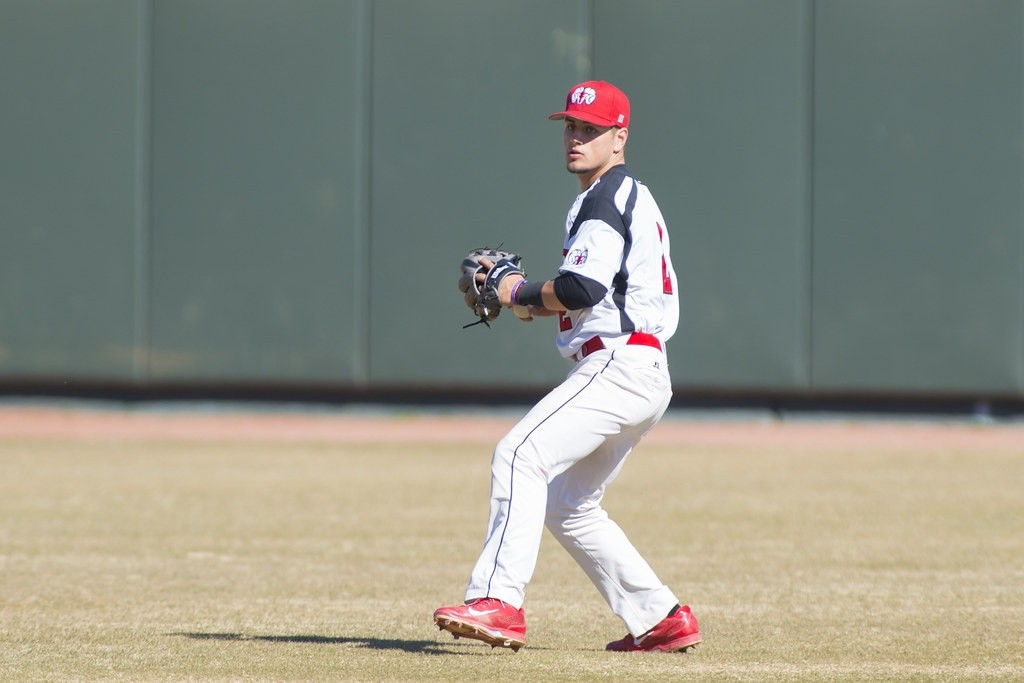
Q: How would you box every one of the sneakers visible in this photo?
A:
[605,604,702,651]
[433,597,526,652]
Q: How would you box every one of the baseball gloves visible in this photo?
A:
[459,248,520,321]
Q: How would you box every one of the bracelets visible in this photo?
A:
[511,280,548,308]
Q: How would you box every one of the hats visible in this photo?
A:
[546,77,630,128]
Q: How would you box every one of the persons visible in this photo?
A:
[434,80,703,655]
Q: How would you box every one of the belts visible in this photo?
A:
[570,333,662,360]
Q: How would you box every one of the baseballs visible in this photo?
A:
[512,304,534,318]
